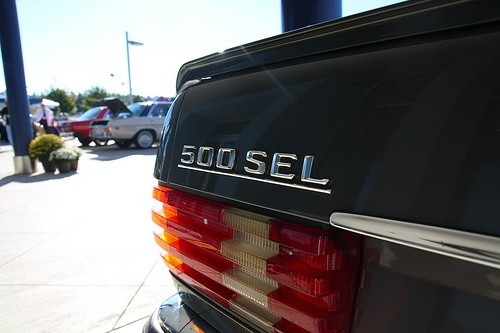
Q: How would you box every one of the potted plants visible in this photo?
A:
[28,134,83,173]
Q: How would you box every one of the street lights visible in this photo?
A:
[125,30,143,105]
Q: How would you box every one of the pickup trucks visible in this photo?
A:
[142,0,500,333]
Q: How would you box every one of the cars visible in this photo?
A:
[57,97,175,149]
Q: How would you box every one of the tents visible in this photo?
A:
[1,92,60,108]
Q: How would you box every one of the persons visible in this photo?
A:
[0,115,13,146]
[34,103,59,140]
